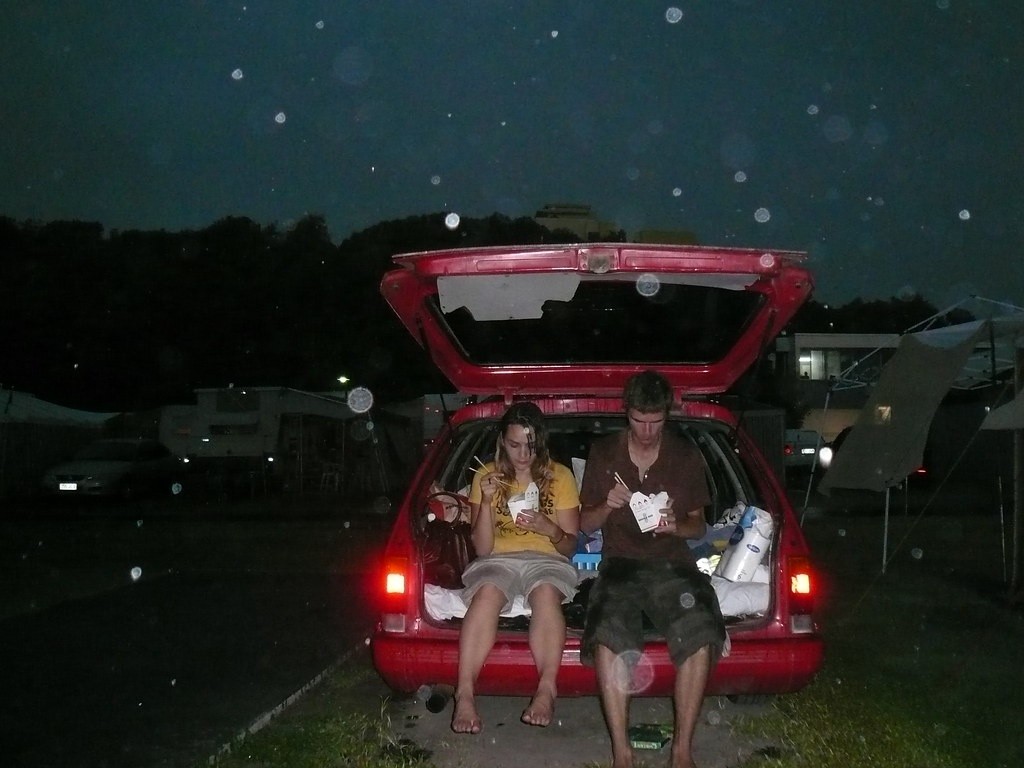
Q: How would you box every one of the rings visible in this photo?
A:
[489,479,491,484]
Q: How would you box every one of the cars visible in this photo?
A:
[365,247,825,715]
[781,428,828,482]
[826,424,928,488]
[42,433,170,503]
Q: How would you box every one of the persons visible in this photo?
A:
[580,371,724,768]
[451,402,580,732]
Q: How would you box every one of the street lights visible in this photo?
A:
[337,376,350,494]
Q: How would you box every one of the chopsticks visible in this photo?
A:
[468,456,517,492]
[614,472,629,490]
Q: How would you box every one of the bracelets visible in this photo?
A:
[550,529,564,544]
